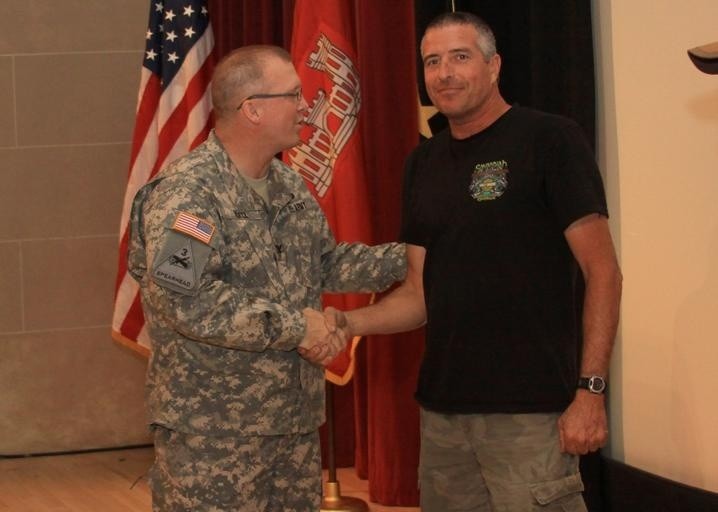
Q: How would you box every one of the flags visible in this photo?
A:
[112,0,220,359]
[283,0,374,385]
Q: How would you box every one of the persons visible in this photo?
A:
[300,12,622,511]
[128,45,406,511]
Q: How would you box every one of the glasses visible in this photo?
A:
[236,88,303,109]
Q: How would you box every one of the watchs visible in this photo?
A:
[576,377,608,396]
